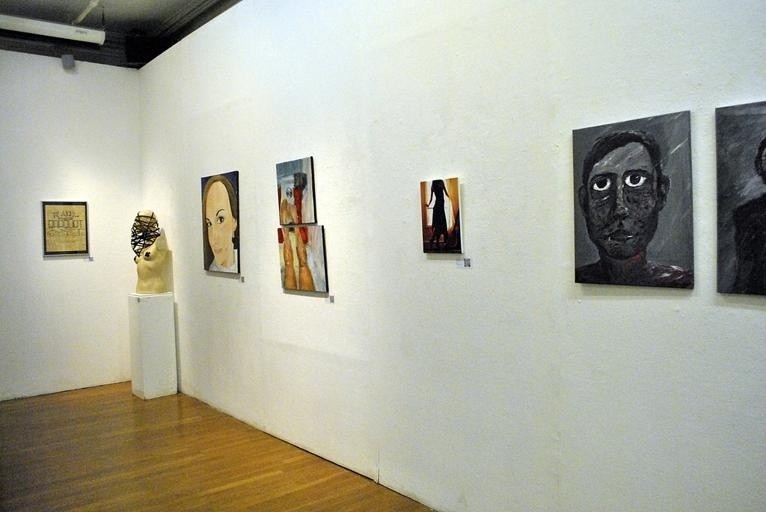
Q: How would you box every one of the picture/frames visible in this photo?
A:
[43,202,89,255]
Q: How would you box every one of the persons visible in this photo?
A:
[201,174,240,273]
[575,126,695,289]
[423,180,451,249]
[279,199,314,292]
